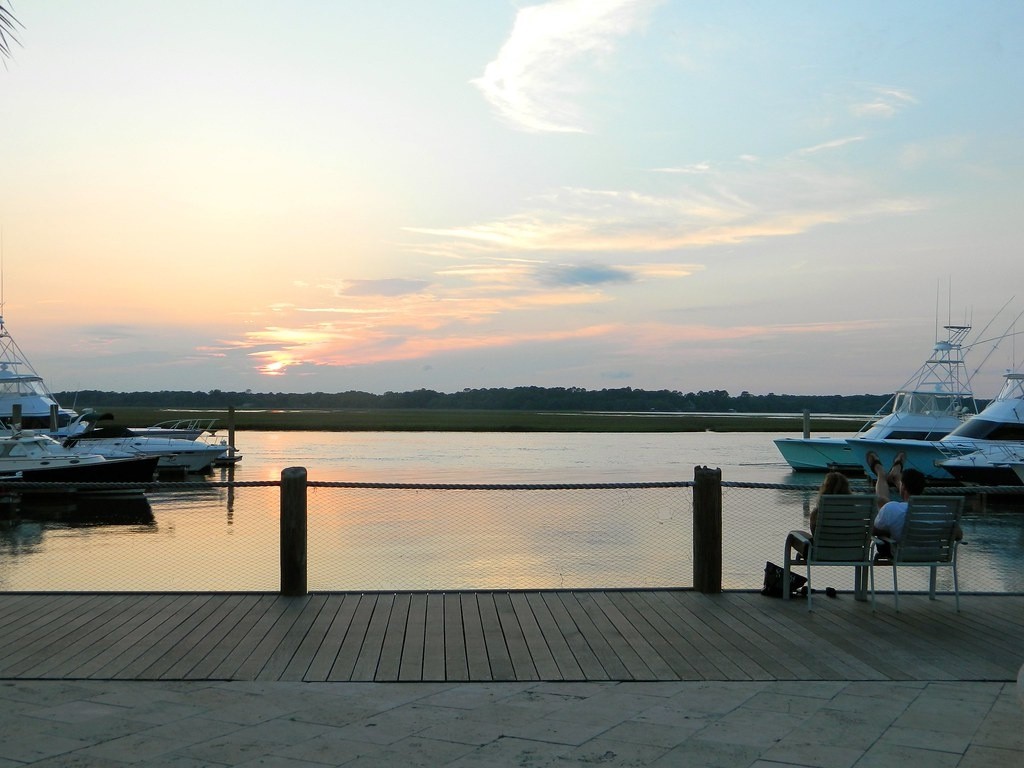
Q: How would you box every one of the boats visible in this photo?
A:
[0,403,179,496]
[772,276,1024,473]
[930,437,1024,489]
[843,370,1024,487]
[0,315,242,477]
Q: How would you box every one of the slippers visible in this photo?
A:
[866,452,883,476]
[889,451,906,474]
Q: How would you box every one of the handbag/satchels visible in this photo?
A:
[760,561,808,599]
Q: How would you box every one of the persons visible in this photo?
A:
[866,450,963,562]
[809,472,867,563]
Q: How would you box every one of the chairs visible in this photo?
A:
[869,495,967,613]
[782,494,879,612]
